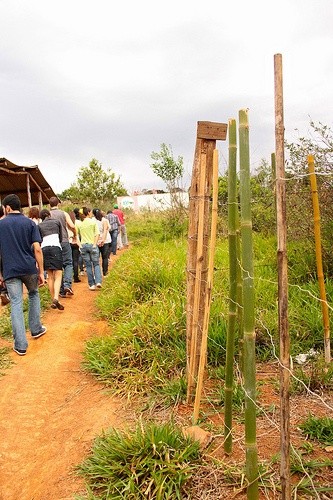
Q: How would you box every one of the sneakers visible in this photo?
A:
[88,285,95,290]
[96,283,102,288]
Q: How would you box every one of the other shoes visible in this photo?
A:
[13,348,27,355]
[58,290,66,297]
[65,288,74,298]
[50,304,57,308]
[30,327,48,338]
[53,299,64,310]
[80,270,84,275]
[74,279,81,283]
[1,291,10,305]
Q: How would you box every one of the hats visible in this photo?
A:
[114,204,118,209]
[78,209,83,215]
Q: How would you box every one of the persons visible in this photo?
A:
[0,197,129,310]
[0,194,48,355]
